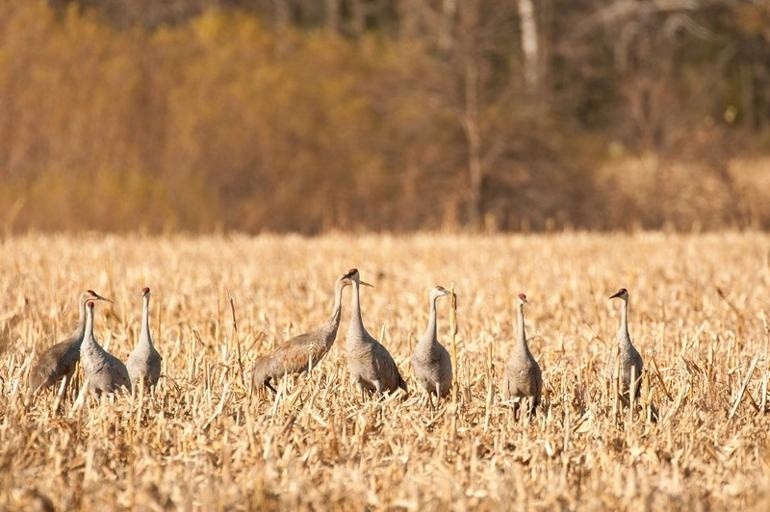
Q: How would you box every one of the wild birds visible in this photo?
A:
[505,293,542,414]
[411,285,451,396]
[251,274,375,395]
[25,287,163,402]
[609,288,644,397]
[342,269,408,398]
